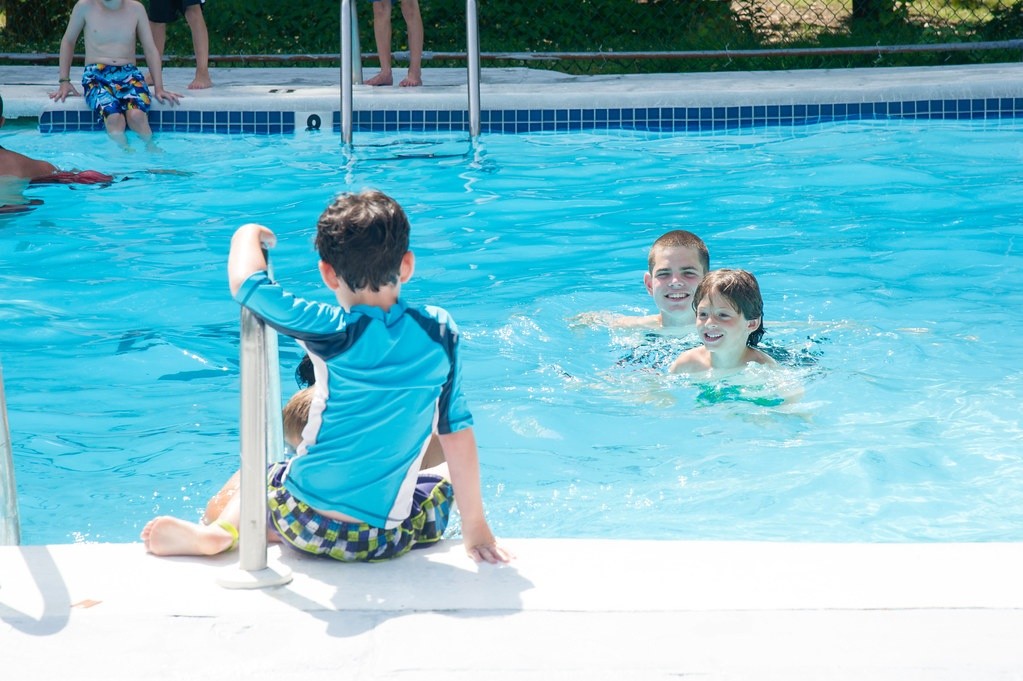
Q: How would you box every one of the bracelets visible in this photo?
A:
[58,77,71,82]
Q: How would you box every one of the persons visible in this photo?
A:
[363,0,424,87]
[0,96,115,214]
[49,0,185,152]
[202,385,316,526]
[140,187,514,564]
[144,0,213,89]
[573,230,871,360]
[669,266,810,425]
